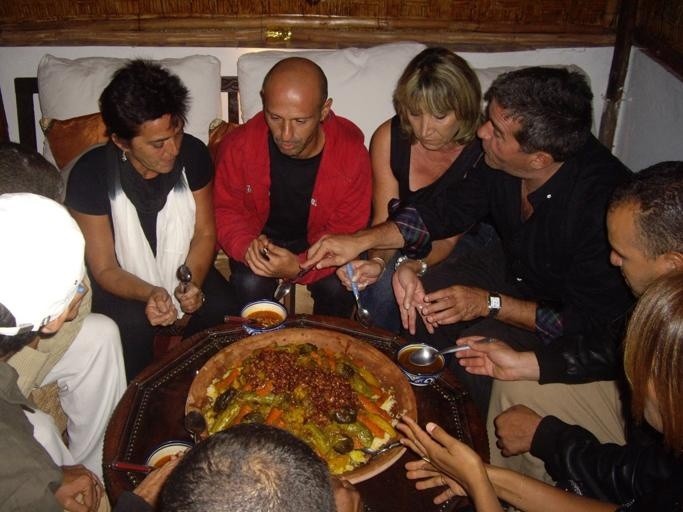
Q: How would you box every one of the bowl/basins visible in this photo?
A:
[397,343,445,386]
[241,298,288,336]
[145,438,196,476]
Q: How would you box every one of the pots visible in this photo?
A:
[185,325,419,487]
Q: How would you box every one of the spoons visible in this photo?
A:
[183,410,205,444]
[272,253,331,300]
[410,336,495,366]
[346,262,372,329]
[176,263,191,294]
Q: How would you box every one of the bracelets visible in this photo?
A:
[365,255,385,274]
[395,255,428,278]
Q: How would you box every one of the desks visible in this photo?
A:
[102,313,490,511]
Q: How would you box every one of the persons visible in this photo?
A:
[0,138,131,486]
[298,63,638,418]
[366,39,497,350]
[0,192,113,511]
[59,59,241,387]
[394,262,681,512]
[112,420,363,510]
[212,54,372,320]
[455,160,681,512]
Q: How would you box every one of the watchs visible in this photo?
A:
[484,286,506,321]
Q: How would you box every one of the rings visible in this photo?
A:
[423,455,435,464]
[259,247,268,254]
[94,482,98,490]
[201,298,205,303]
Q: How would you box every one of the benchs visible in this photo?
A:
[14,75,295,311]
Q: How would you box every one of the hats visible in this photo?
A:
[1,193,86,336]
